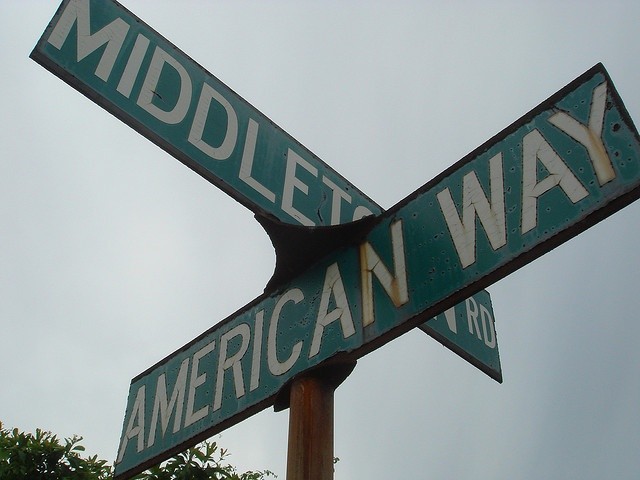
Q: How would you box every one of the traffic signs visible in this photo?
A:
[30,1,502,383]
[114,61,640,480]
